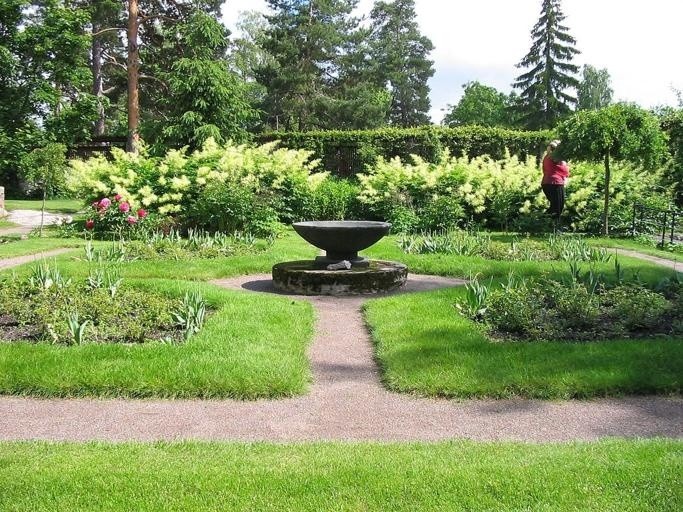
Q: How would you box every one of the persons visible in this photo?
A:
[541,138,568,221]
